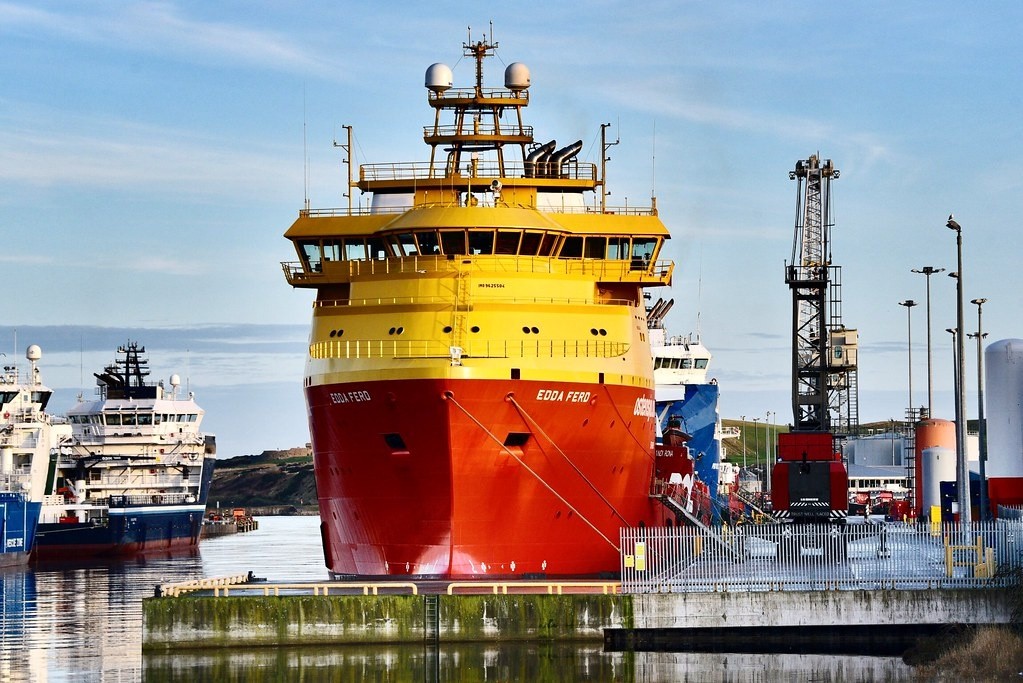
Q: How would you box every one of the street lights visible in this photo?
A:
[966,298,988,532]
[912,266,946,418]
[899,299,921,421]
[945,214,980,544]
[740,411,777,470]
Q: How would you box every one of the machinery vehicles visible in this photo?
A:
[768,148,858,563]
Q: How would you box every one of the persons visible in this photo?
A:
[788,423,793,433]
[863,500,870,524]
[878,521,888,551]
[75,455,85,480]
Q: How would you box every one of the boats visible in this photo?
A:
[643,294,741,529]
[1,324,54,570]
[281,21,678,580]
[34,339,219,561]
